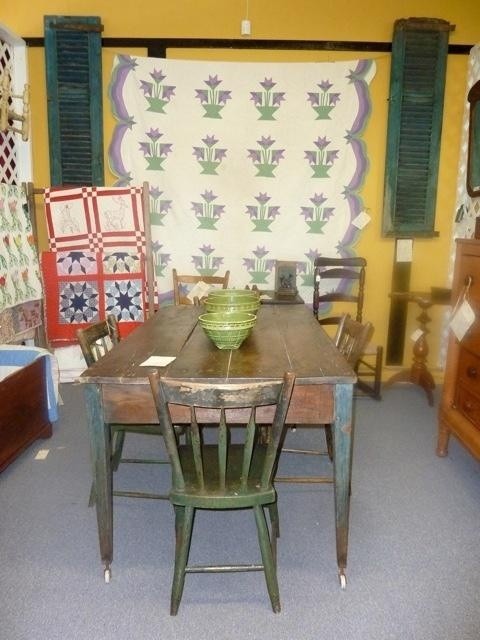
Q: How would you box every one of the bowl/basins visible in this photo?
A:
[208,289,258,298]
[202,296,261,316]
[198,312,257,350]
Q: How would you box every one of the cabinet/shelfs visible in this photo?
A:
[0,345,55,476]
[436,238,480,466]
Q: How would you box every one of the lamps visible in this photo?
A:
[241,2,251,37]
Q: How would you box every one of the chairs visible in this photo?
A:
[75,314,192,507]
[312,257,384,403]
[258,311,375,486]
[172,269,231,306]
[147,365,297,618]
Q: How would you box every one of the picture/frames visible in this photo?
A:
[467,80,480,201]
[275,261,297,300]
